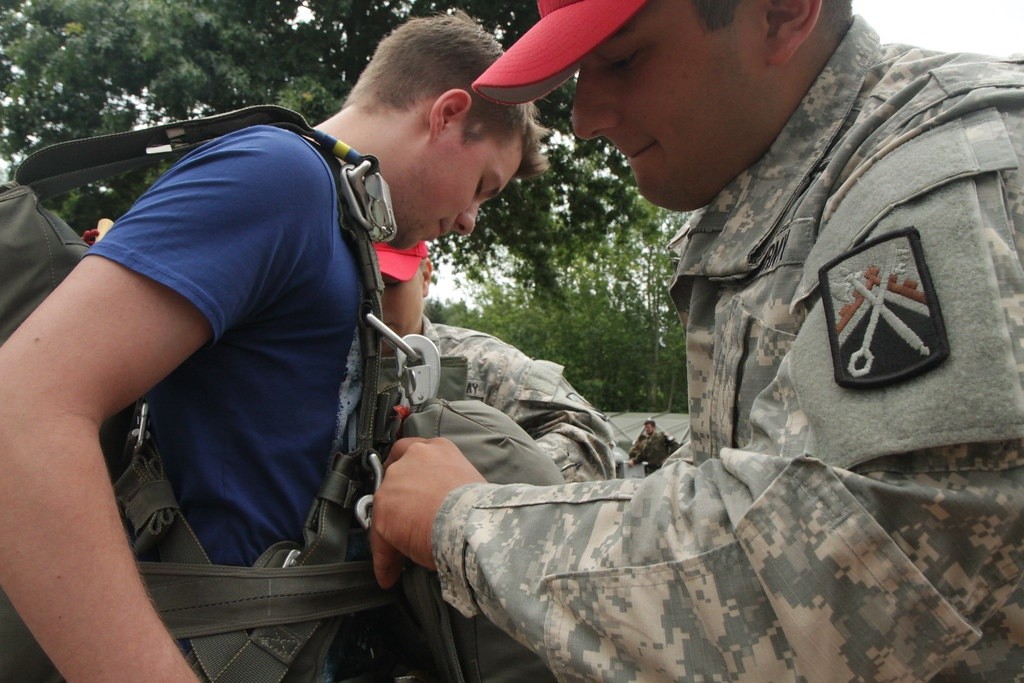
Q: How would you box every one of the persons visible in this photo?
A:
[623,419,682,474]
[368,236,614,485]
[1,0,543,683]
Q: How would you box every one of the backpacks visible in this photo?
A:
[0,102,401,683]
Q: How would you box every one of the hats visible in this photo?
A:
[370,238,428,281]
[471,0,648,105]
